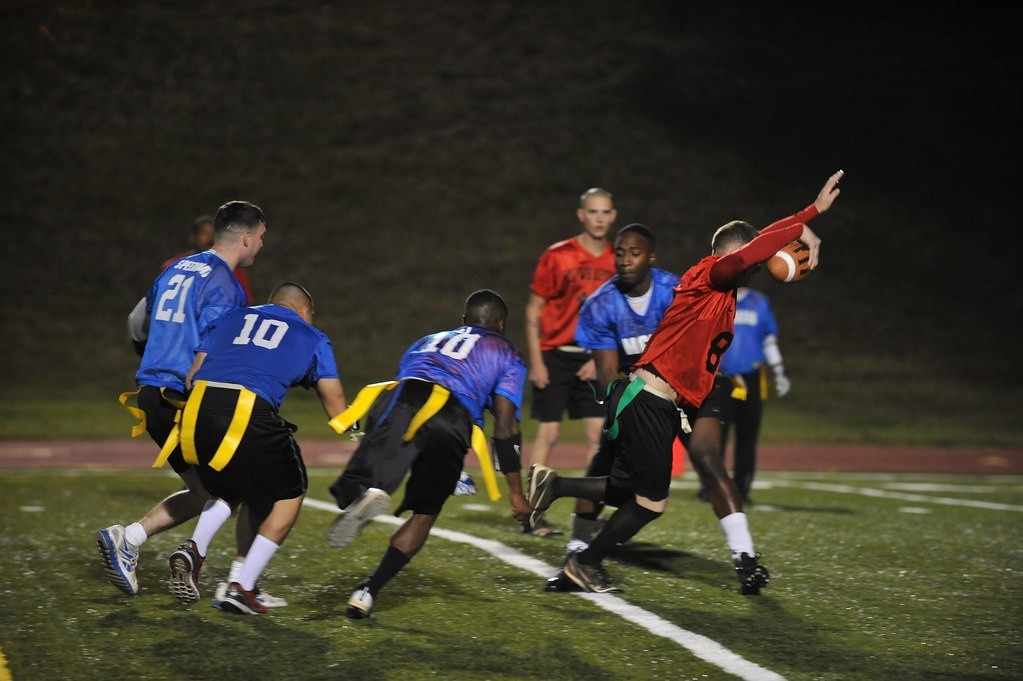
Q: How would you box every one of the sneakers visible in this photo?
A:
[96,524,139,596]
[216,582,268,615]
[168,539,206,605]
[210,582,287,611]
[565,539,588,555]
[544,570,585,592]
[524,463,557,532]
[562,550,625,594]
[328,488,391,550]
[734,553,770,588]
[346,586,373,620]
[520,517,562,538]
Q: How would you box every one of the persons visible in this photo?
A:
[163,218,254,307]
[691,275,789,503]
[529,168,844,593]
[526,187,616,531]
[329,291,531,620]
[168,283,359,616]
[95,201,288,608]
[545,225,770,594]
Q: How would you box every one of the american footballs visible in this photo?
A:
[767,240,810,283]
